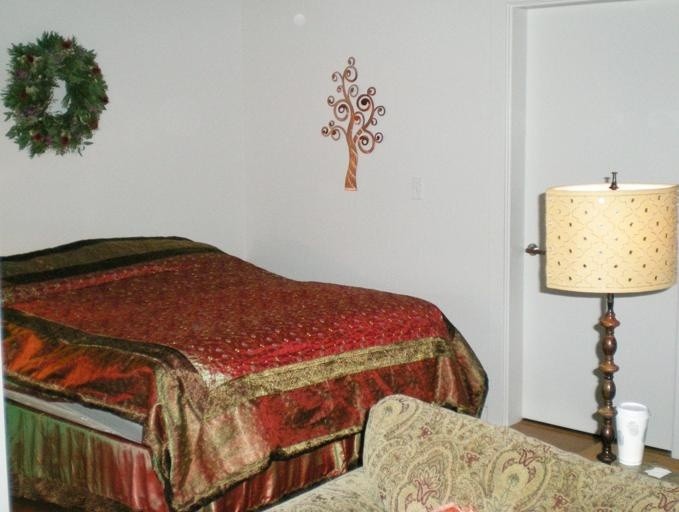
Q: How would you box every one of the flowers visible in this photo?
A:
[1,31,110,159]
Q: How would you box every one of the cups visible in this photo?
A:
[615,401,651,468]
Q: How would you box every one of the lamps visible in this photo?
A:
[524,171,679,464]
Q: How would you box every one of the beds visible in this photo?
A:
[1,236,489,512]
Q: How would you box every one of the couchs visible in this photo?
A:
[262,393,678,511]
